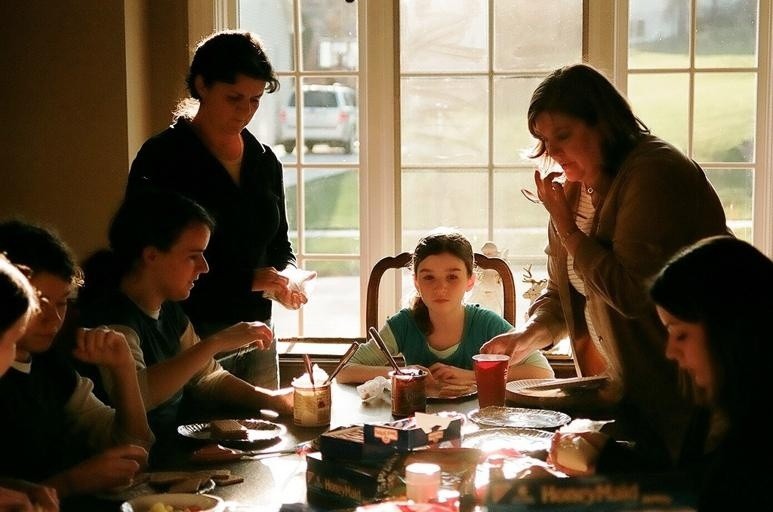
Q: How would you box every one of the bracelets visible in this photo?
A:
[560,226,581,245]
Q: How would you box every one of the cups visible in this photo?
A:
[405,462,441,502]
[472,353,509,407]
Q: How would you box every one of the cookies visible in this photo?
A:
[439,384,473,398]
[148,468,244,494]
[211,417,248,441]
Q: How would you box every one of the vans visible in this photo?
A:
[278,84,356,154]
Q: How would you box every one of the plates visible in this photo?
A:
[425,382,477,400]
[176,418,285,442]
[461,405,572,451]
[505,376,606,399]
[100,472,223,512]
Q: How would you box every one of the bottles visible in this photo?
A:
[489,455,506,482]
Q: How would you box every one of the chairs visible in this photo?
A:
[366,253,516,343]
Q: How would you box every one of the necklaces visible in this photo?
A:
[584,182,600,195]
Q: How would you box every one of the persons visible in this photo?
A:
[481,64,727,407]
[547,237,773,509]
[66,195,304,420]
[2,222,157,497]
[337,228,555,394]
[121,29,317,389]
[2,258,146,509]
